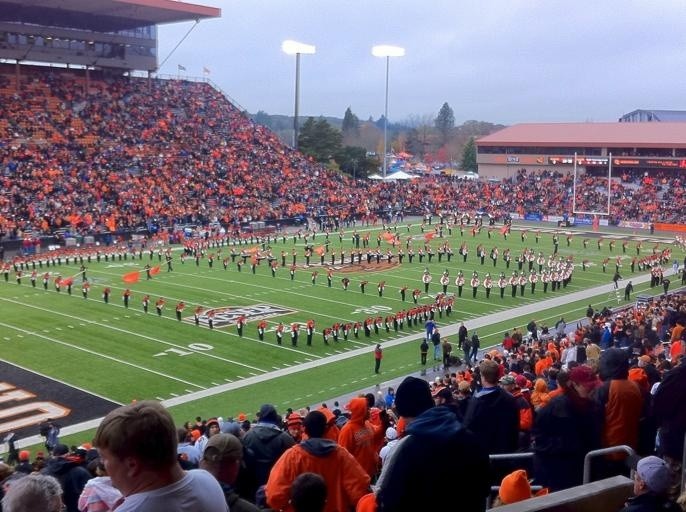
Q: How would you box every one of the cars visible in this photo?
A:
[467,171,475,180]
[434,164,441,170]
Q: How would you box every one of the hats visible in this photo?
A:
[498,375,513,387]
[435,388,449,403]
[636,355,652,365]
[204,417,220,430]
[570,367,604,393]
[201,434,242,461]
[392,375,432,417]
[637,456,671,493]
[490,349,498,361]
[385,427,397,440]
[258,403,280,427]
[52,443,69,457]
[515,375,529,387]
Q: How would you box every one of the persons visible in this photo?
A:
[1,72,685,512]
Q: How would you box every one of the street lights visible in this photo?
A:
[280,38,318,146]
[369,43,408,176]
